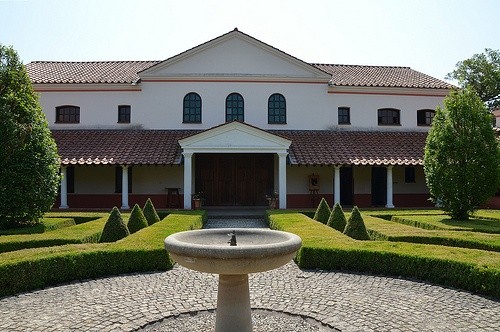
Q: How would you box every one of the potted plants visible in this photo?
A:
[192,192,205,210]
[265,191,279,210]
